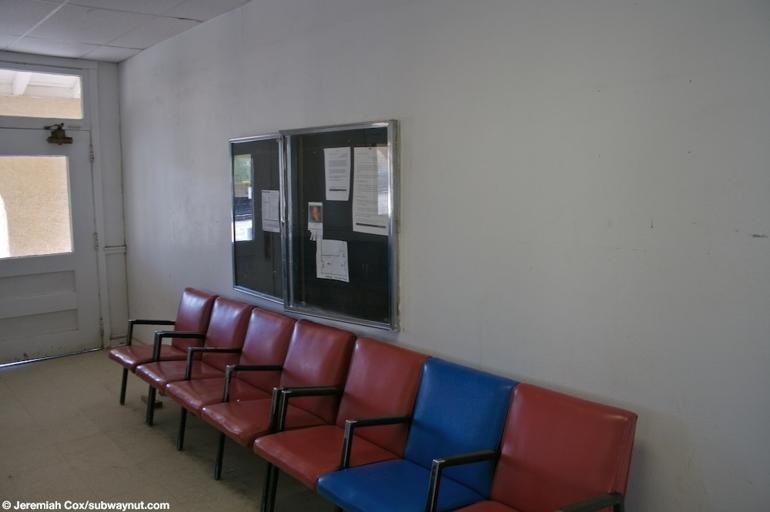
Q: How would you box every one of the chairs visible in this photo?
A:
[108,287,641,511]
